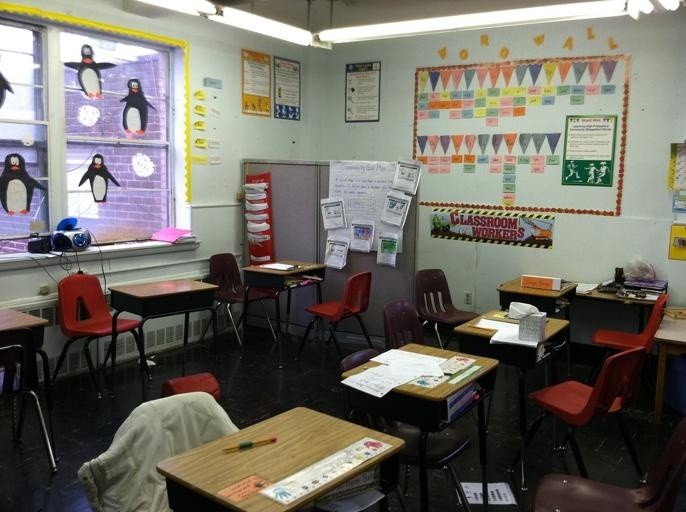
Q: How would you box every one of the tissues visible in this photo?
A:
[508,301,547,342]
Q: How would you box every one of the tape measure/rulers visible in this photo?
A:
[446,365,484,385]
[265,437,394,506]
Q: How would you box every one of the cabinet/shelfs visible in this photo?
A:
[240,158,416,345]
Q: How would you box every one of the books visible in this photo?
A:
[556,298,570,313]
[311,466,387,512]
[151,227,197,245]
[615,277,668,301]
[447,381,475,418]
[244,183,302,273]
[575,281,599,294]
[456,480,519,506]
[474,310,551,347]
[320,160,422,271]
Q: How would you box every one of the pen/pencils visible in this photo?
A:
[222,436,277,451]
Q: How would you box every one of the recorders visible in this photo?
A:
[52,227,91,252]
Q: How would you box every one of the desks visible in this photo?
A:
[652,304,686,423]
[107,279,219,400]
[452,310,571,493]
[339,342,500,512]
[571,282,667,333]
[496,277,578,389]
[0,307,50,445]
[156,406,407,511]
[241,260,328,369]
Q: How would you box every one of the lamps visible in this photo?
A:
[318,1,628,45]
[206,1,314,47]
[137,0,220,18]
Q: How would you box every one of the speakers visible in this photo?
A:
[28,236,52,253]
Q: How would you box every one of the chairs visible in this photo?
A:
[511,345,650,484]
[79,391,240,512]
[198,252,278,359]
[382,299,424,350]
[414,268,479,347]
[587,294,667,387]
[0,343,59,474]
[51,273,153,400]
[530,415,685,512]
[341,347,474,512]
[295,271,372,363]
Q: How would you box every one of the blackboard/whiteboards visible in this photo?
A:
[412,56,628,217]
